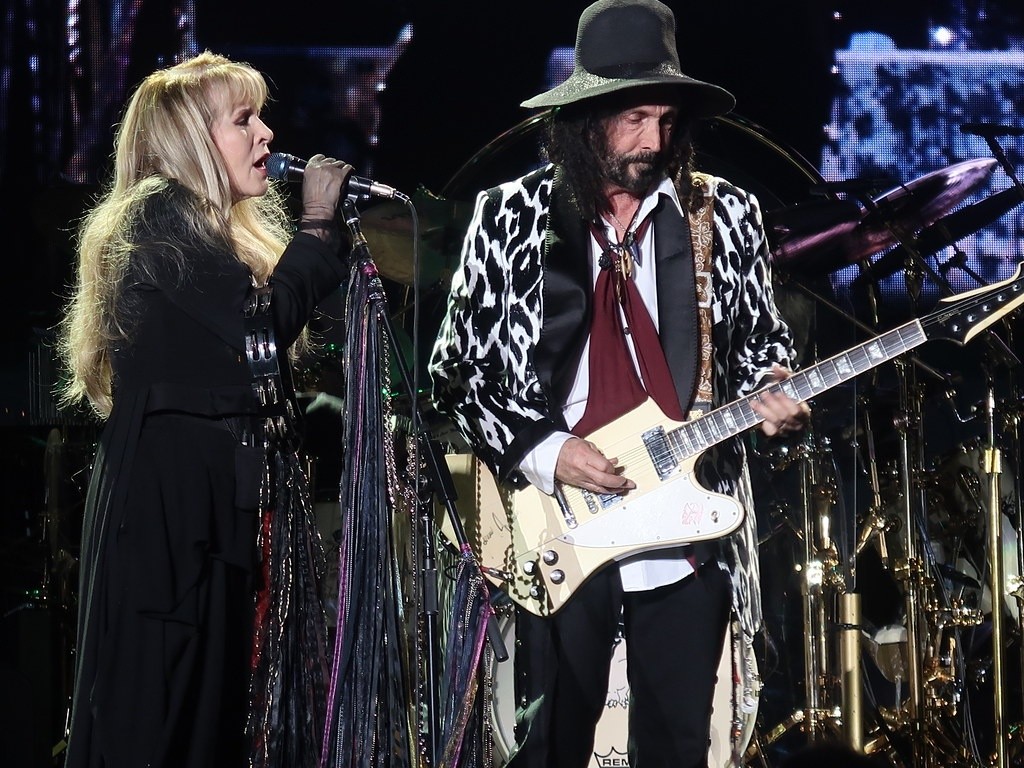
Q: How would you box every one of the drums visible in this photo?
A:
[459,548,768,768]
[307,486,429,653]
[903,430,1024,630]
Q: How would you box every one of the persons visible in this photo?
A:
[427,0,813,768]
[62,53,352,766]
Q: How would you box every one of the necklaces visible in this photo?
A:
[604,202,643,280]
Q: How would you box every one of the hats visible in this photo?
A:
[520,0,736,118]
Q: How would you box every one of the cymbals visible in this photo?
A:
[773,154,1000,278]
[846,182,1024,303]
[358,195,462,293]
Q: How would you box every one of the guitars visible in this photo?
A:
[429,258,1024,621]
